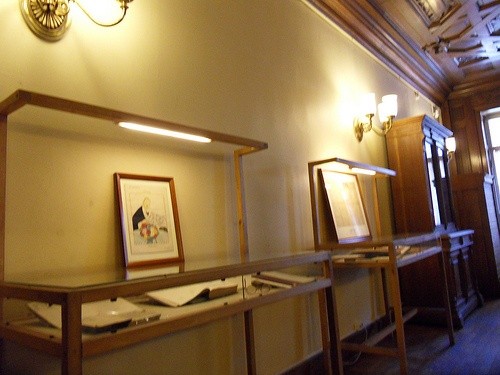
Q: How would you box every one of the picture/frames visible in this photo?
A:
[319,168,373,245]
[113,172,185,270]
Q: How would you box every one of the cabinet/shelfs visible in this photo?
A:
[0,89,456,375]
[385,114,485,328]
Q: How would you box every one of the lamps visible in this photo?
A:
[445,137,456,162]
[20,1,135,42]
[352,94,398,143]
